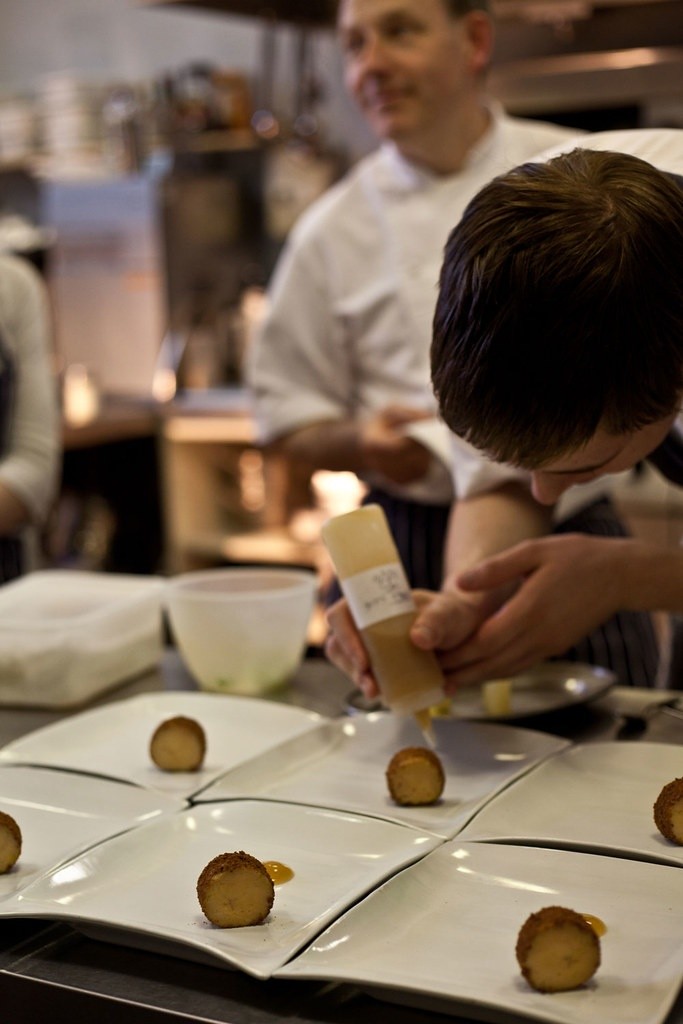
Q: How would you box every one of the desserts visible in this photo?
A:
[515,906,601,993]
[385,746,444,806]
[149,715,207,772]
[196,850,275,928]
[652,776,683,847]
[0,811,23,875]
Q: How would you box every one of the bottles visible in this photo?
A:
[320,505,447,753]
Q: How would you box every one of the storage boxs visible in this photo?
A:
[0,570,162,711]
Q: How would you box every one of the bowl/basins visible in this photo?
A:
[166,567,317,692]
[0,568,165,706]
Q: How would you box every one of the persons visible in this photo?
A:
[251,0,662,685]
[324,129,683,698]
[0,255,56,588]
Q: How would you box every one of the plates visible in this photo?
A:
[193,711,573,840]
[271,841,683,1024]
[0,690,332,798]
[0,800,445,981]
[452,741,683,865]
[339,652,623,716]
[0,767,191,903]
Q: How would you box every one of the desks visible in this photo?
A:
[0,646,682,1024]
